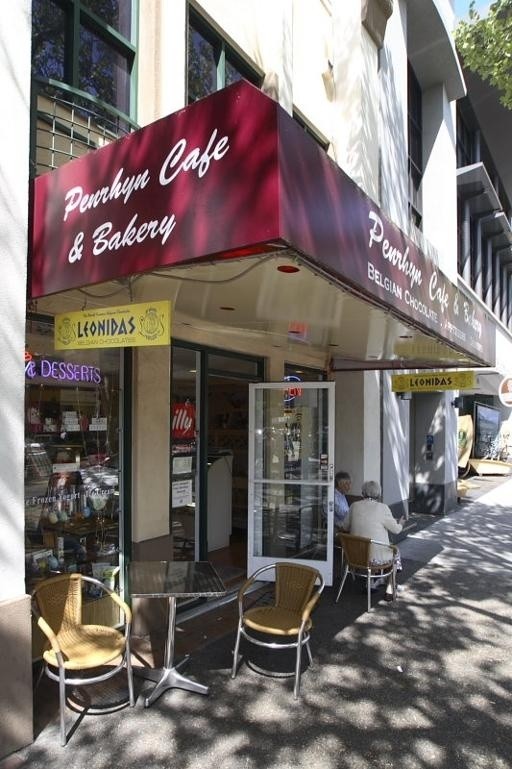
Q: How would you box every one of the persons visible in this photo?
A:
[321,470,352,530]
[342,479,407,605]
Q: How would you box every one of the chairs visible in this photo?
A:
[29,573,134,748]
[336,531,399,614]
[229,561,325,700]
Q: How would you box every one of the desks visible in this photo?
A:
[126,561,227,709]
